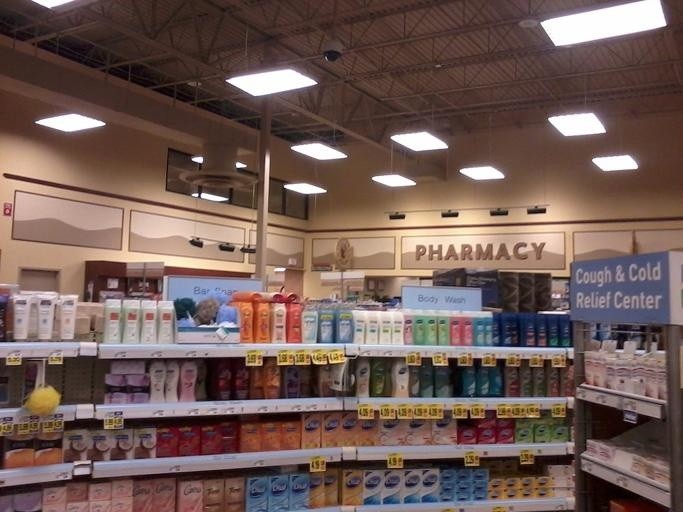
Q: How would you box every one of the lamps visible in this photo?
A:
[386,205,547,222]
[188,234,256,254]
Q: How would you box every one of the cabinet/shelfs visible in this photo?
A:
[568,249,682,511]
[1,341,575,512]
